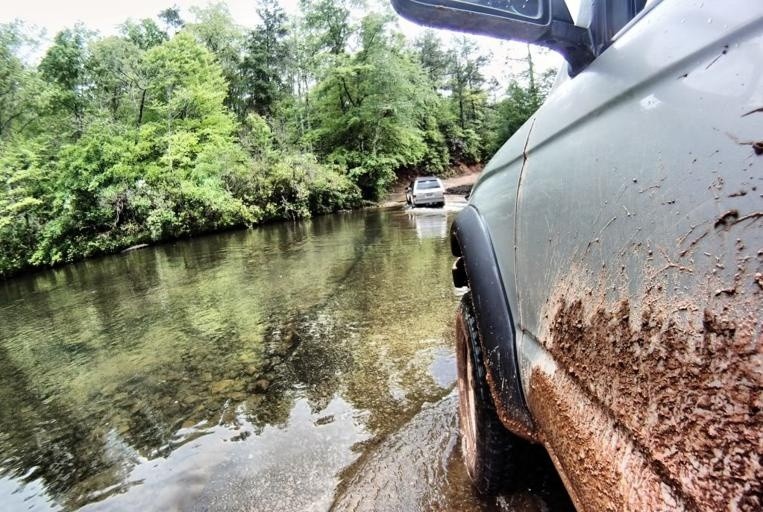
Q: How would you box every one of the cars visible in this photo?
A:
[391,0,760,512]
[404,176,445,207]
